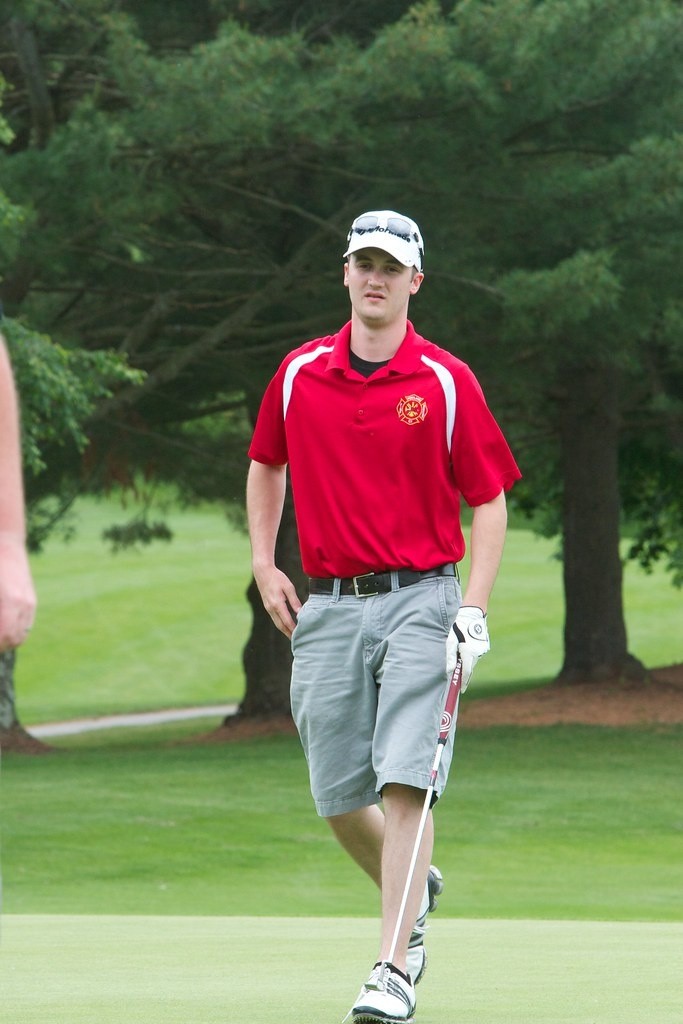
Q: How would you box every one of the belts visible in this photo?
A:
[308,560,457,599]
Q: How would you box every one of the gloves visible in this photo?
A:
[446,605,490,694]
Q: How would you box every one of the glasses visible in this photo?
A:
[347,216,425,272]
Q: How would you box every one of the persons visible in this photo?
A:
[0,334,36,649]
[245,209,523,1024]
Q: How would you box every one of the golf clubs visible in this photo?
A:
[365,651,466,993]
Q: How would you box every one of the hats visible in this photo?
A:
[343,210,426,272]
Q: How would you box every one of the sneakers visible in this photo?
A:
[350,960,416,1024]
[406,861,446,985]
